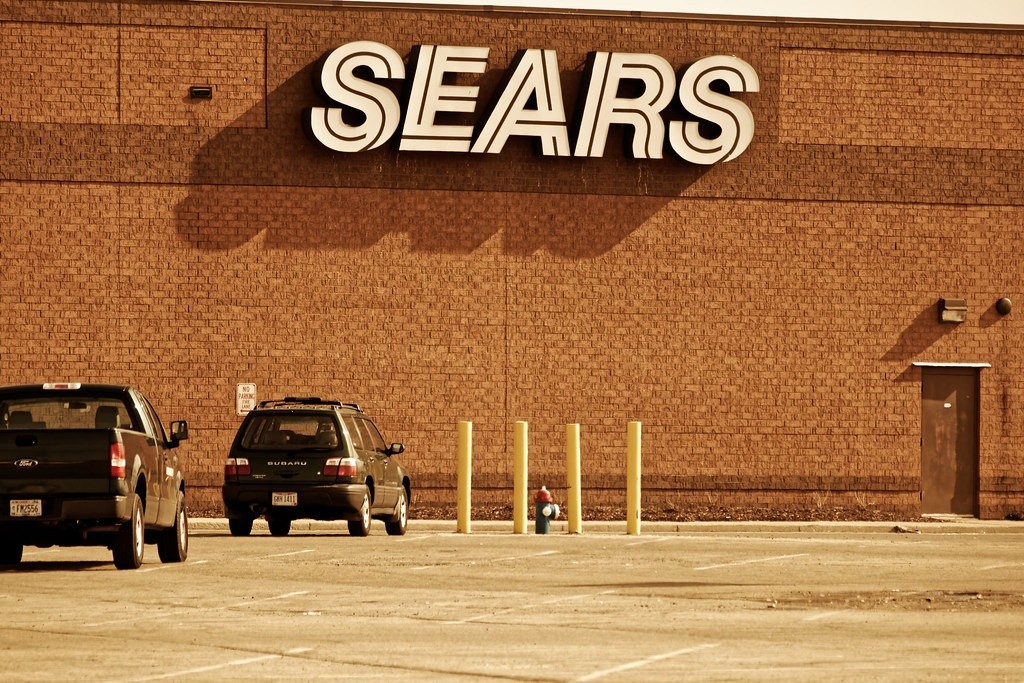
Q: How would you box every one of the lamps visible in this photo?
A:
[938,297,968,324]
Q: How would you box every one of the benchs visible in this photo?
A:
[252,431,338,448]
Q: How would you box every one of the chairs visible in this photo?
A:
[7,412,46,429]
[94,405,120,429]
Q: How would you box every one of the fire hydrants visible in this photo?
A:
[533,485,560,535]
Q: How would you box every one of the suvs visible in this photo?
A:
[220,396,411,537]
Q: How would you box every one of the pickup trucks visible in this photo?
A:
[0,382,190,568]
